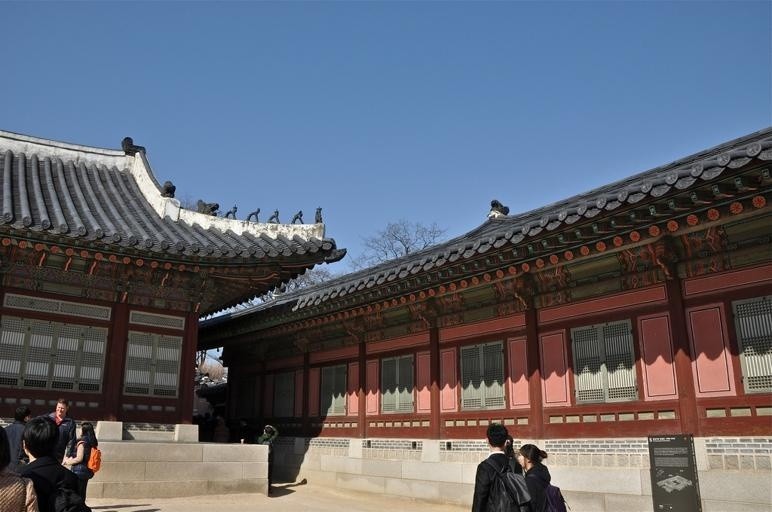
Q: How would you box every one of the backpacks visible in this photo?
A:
[526,473,567,512]
[77,440,102,473]
[22,467,92,511]
[483,455,533,512]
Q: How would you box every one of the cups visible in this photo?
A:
[241,439,244,444]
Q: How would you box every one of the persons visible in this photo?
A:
[4,405,33,474]
[502,434,523,476]
[470,423,532,511]
[49,397,77,466]
[62,421,98,504]
[194,411,256,444]
[20,414,90,511]
[517,443,566,511]
[258,424,279,494]
[0,423,40,512]
